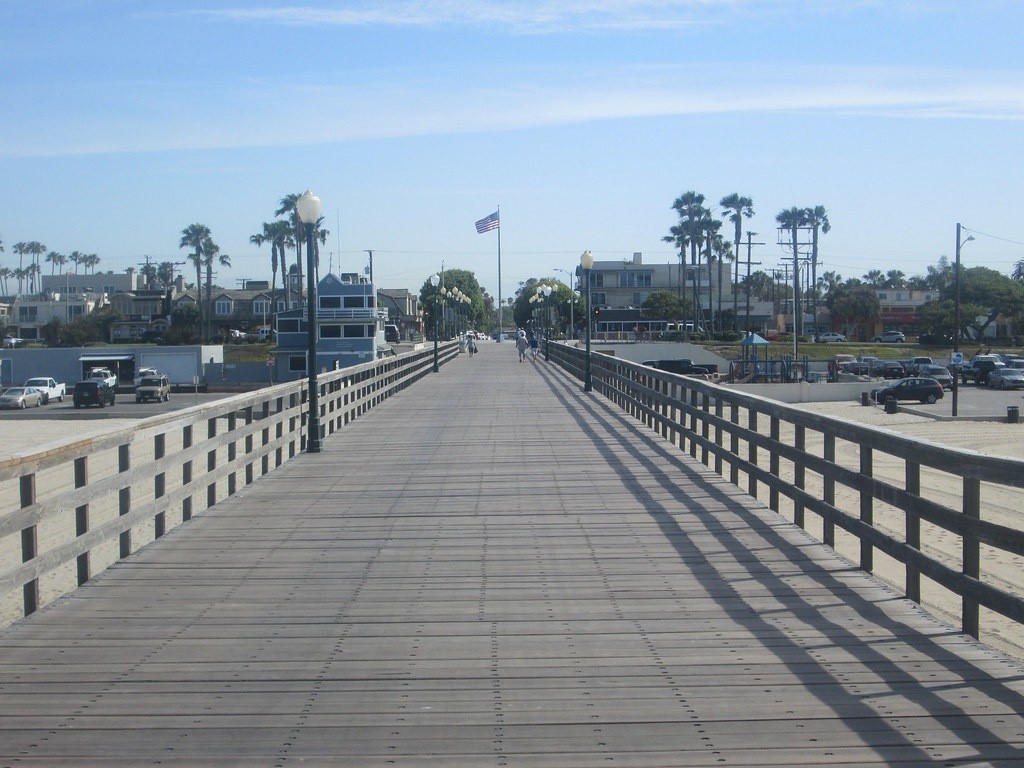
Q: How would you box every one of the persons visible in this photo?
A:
[516,327,528,363]
[465,336,476,357]
[531,336,539,362]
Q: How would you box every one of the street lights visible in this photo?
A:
[295,186,325,453]
[580,250,593,391]
[951,235,975,417]
[554,268,574,341]
[452,286,472,339]
[429,273,440,374]
[440,285,446,341]
[529,284,550,361]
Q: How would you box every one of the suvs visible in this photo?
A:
[384,324,401,344]
[73,378,115,409]
[135,374,171,404]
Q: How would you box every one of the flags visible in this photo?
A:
[475,211,499,234]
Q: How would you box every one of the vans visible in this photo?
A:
[666,323,706,334]
[257,328,277,342]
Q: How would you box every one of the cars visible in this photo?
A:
[460,330,494,341]
[86,366,117,390]
[2,334,23,349]
[870,377,945,405]
[871,330,906,343]
[133,368,159,388]
[828,354,859,372]
[764,332,793,342]
[0,386,43,409]
[843,354,1024,392]
[819,332,847,342]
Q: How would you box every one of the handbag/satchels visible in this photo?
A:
[474,347,478,353]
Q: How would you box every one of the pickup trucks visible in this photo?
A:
[23,376,66,405]
